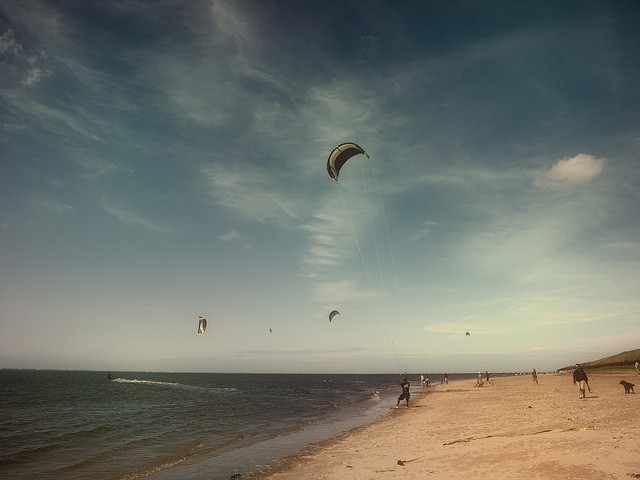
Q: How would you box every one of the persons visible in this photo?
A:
[485,370,490,381]
[531,368,539,385]
[444,373,448,385]
[475,372,483,387]
[420,374,431,387]
[573,363,590,399]
[394,378,410,408]
